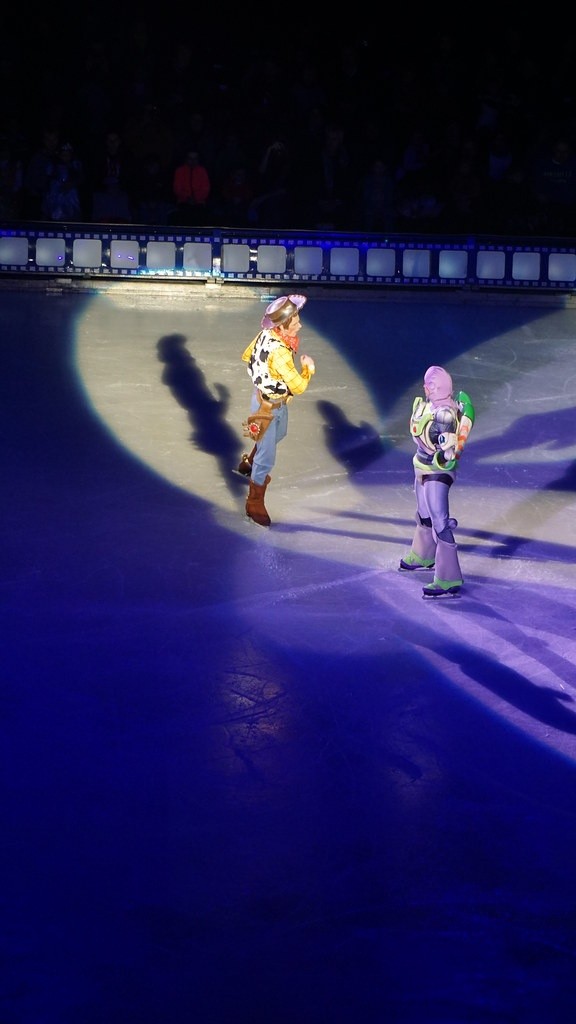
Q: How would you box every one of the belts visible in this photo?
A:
[256,389,288,409]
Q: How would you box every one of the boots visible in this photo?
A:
[398,510,438,571]
[231,454,271,485]
[245,474,272,530]
[422,534,464,600]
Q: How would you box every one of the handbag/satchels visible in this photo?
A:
[242,400,275,442]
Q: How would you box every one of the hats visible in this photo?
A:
[261,294,307,330]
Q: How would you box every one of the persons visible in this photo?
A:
[398,365,475,601]
[237,293,315,527]
[0,31,576,240]
